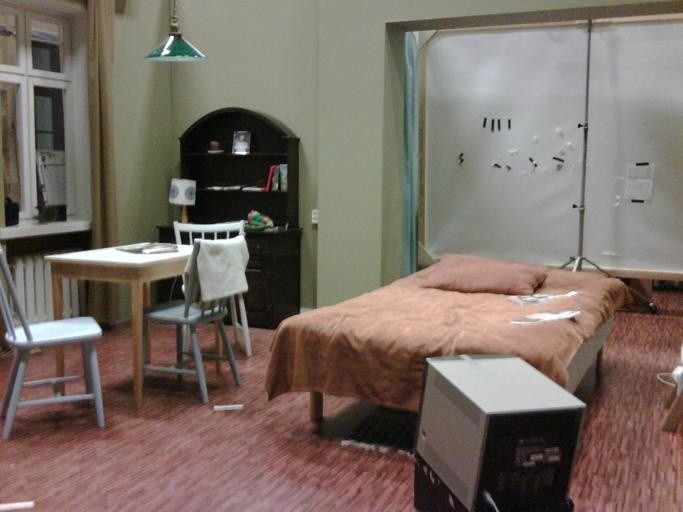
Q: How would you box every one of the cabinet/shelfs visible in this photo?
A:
[179,107,303,330]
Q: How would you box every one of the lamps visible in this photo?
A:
[144,0,206,63]
[169,177,197,223]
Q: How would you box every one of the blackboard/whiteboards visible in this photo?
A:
[418,13,683,282]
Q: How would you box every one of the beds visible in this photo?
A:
[265,258,632,433]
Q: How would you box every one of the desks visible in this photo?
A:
[44,241,222,408]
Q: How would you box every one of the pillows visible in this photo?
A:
[420,255,549,294]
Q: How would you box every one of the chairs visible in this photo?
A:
[146,234,247,405]
[173,220,252,358]
[0,248,105,442]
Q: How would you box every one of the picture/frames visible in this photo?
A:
[232,130,251,155]
